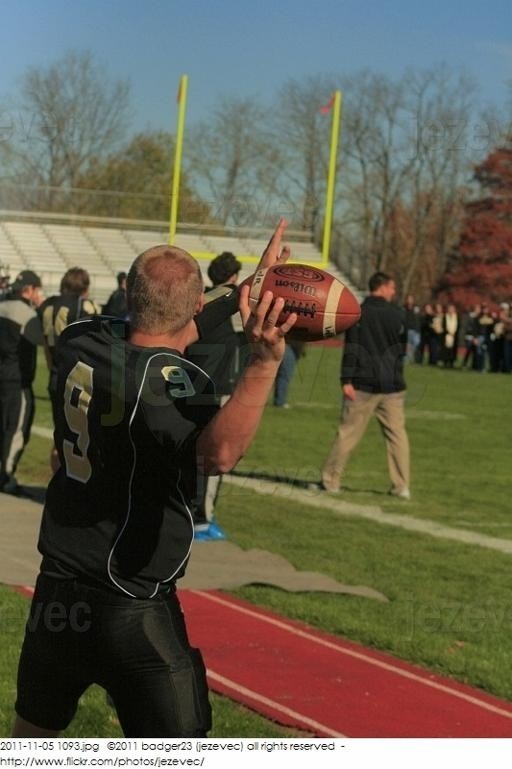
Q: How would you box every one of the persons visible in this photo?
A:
[2,216,512,740]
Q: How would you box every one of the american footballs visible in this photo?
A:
[239,264,363,342]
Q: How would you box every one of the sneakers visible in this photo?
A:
[307,480,337,493]
[389,486,410,499]
